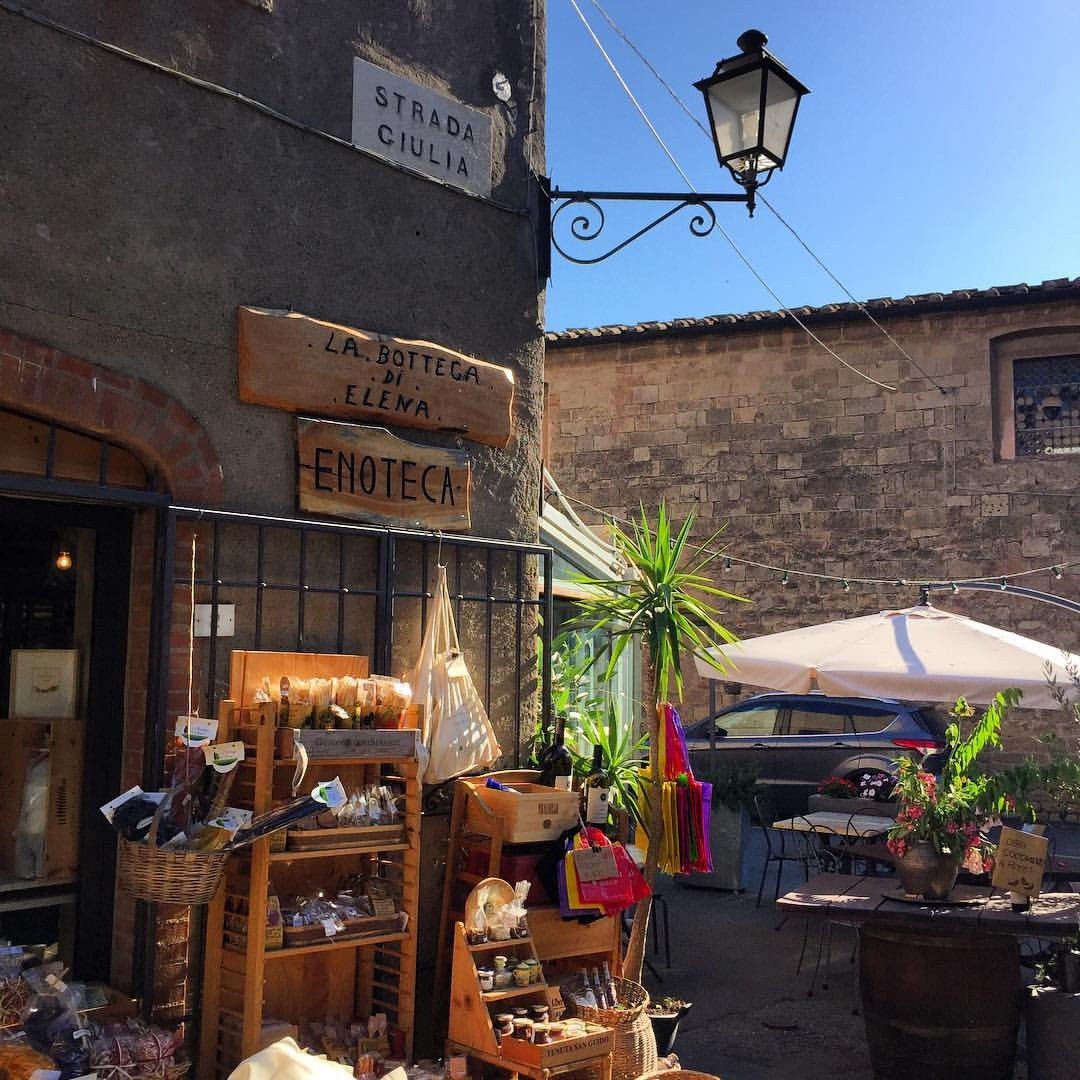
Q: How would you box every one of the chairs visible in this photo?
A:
[754,794,1056,995]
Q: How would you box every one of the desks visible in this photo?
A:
[773,811,895,929]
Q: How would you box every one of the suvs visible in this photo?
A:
[682,690,963,815]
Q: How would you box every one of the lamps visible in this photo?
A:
[538,30,812,279]
[56,528,71,570]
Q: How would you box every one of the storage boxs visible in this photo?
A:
[470,845,559,907]
[10,650,81,722]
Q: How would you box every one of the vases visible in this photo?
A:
[895,839,958,897]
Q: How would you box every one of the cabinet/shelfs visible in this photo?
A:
[196,650,620,1080]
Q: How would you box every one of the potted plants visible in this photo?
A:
[558,496,756,1057]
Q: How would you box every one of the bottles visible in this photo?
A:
[601,961,618,1006]
[582,745,609,835]
[485,778,517,791]
[591,967,609,1010]
[581,967,598,1011]
[537,716,574,790]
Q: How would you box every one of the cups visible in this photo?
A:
[1010,890,1033,911]
[477,953,608,1045]
[487,903,511,941]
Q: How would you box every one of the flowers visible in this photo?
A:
[816,684,1080,874]
